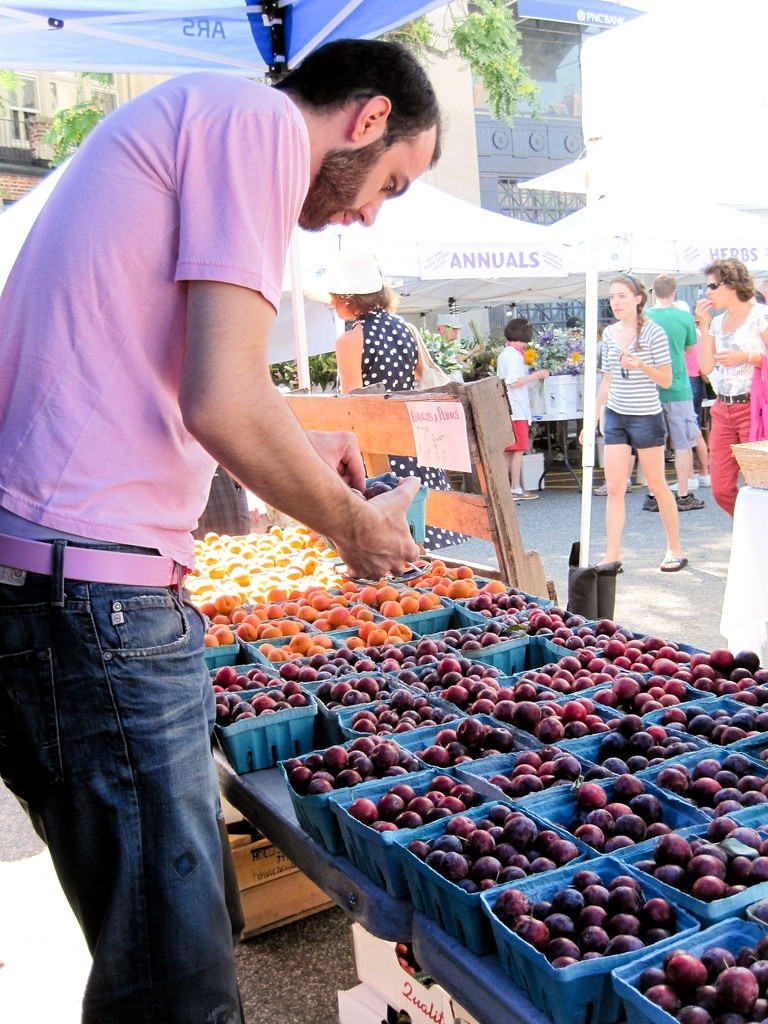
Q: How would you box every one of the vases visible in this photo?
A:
[528,373,602,415]
[521,452,545,491]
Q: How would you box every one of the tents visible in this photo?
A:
[267,176,631,566]
[544,186,768,278]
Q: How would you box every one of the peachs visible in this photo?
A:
[183,523,508,667]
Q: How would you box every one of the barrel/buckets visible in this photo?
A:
[521,452,546,491]
[596,436,604,469]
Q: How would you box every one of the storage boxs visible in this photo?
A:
[185,527,767,1024]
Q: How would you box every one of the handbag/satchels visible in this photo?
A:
[407,322,450,389]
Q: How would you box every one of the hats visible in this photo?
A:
[325,257,381,295]
[437,314,464,329]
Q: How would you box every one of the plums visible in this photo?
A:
[214,588,768,1024]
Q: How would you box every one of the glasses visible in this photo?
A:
[706,281,726,290]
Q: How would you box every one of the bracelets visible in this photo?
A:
[748,353,753,364]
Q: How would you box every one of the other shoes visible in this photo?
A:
[512,490,540,501]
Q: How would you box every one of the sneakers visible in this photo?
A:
[669,479,697,490]
[642,496,659,511]
[695,473,711,487]
[675,493,705,510]
[594,483,607,495]
[626,484,632,493]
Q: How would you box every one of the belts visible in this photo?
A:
[0,533,187,587]
[717,393,749,403]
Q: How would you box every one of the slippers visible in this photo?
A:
[661,558,688,572]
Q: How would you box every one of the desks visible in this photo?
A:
[719,485,768,671]
[530,411,586,494]
[209,739,555,1024]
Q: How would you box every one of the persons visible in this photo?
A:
[0,39,425,1023]
[497,258,768,572]
[325,288,473,550]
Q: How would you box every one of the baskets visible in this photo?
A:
[201,564,766,1024]
[363,471,430,545]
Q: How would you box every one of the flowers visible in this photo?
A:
[268,321,606,396]
[523,421,547,455]
